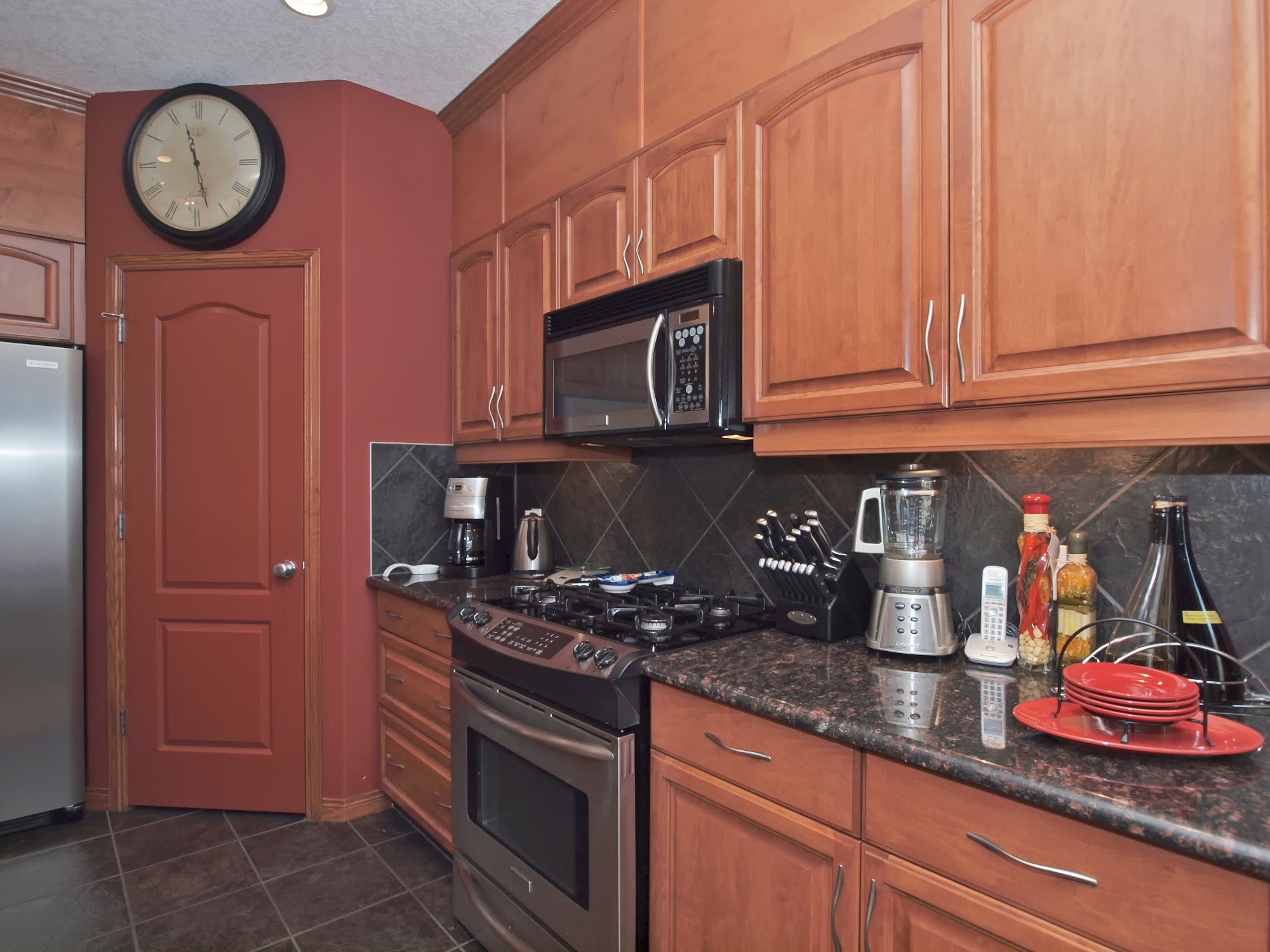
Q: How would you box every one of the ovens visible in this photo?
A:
[447,663,653,952]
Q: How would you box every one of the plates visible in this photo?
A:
[1013,660,1265,760]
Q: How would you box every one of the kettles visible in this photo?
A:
[511,512,558,579]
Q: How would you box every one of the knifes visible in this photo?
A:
[751,506,844,606]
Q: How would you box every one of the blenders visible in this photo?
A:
[869,660,966,756]
[853,459,959,656]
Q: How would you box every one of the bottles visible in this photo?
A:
[1010,481,1248,706]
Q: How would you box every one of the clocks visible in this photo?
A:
[122,81,286,251]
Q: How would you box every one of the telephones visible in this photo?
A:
[961,566,1020,667]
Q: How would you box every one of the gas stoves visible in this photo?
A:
[445,566,773,734]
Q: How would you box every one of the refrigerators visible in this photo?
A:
[0,336,88,834]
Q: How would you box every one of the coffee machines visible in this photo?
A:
[439,473,514,579]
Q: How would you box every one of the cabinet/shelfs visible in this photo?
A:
[0,230,75,347]
[561,104,745,302]
[741,1,1267,425]
[641,682,1270,952]
[444,192,552,443]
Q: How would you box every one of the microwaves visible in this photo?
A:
[541,254,755,452]
